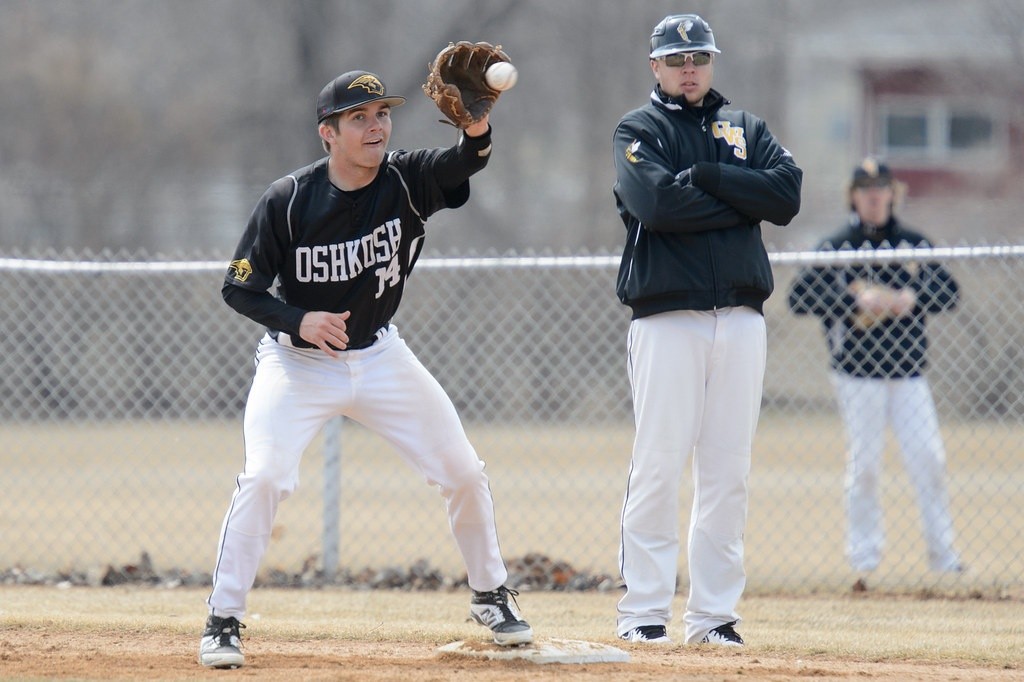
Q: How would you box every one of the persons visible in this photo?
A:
[613,14,803,644]
[786,156,962,595]
[198,40,534,667]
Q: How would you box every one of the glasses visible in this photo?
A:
[856,179,891,188]
[653,51,715,66]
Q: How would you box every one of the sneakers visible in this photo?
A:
[199,616,246,669]
[701,621,745,648]
[470,585,534,647]
[621,625,670,644]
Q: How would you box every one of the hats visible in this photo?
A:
[317,70,408,124]
[649,14,721,58]
[854,157,893,179]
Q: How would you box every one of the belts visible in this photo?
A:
[266,323,389,351]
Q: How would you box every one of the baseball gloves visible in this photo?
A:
[420,41,514,130]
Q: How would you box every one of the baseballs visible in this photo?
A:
[485,61,519,93]
